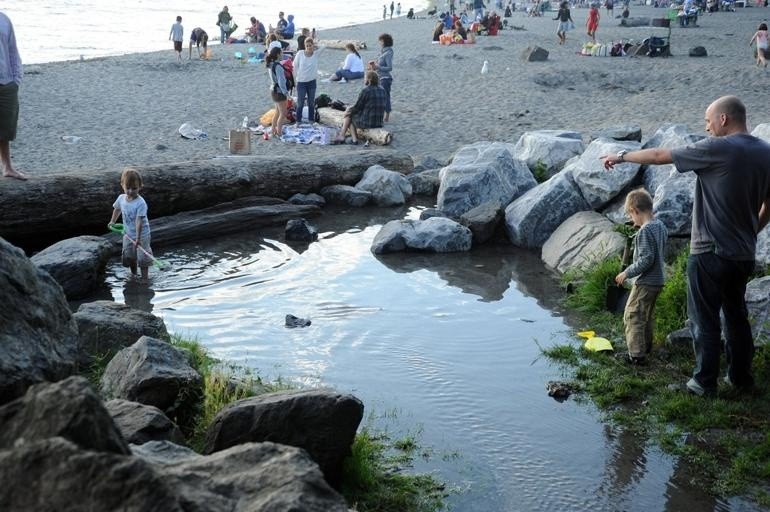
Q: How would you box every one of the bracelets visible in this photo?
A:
[617,149,628,163]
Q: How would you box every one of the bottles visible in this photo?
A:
[241,115,248,131]
[481,59,489,75]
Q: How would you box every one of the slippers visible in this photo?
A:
[345,137,358,145]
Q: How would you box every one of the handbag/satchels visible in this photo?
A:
[315,94,346,111]
[228,126,251,155]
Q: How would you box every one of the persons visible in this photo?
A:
[0,11,28,181]
[292,37,327,128]
[615,185,669,364]
[330,70,387,144]
[383,1,402,22]
[215,5,294,44]
[320,43,365,83]
[266,47,292,138]
[109,169,153,279]
[368,33,394,122]
[428,0,518,44]
[750,23,770,68]
[526,0,630,45]
[407,8,418,20]
[169,16,183,62]
[603,95,770,399]
[188,27,208,60]
[264,28,316,59]
[677,1,697,28]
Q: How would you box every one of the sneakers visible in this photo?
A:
[668,384,694,394]
[718,377,732,387]
[614,352,644,364]
